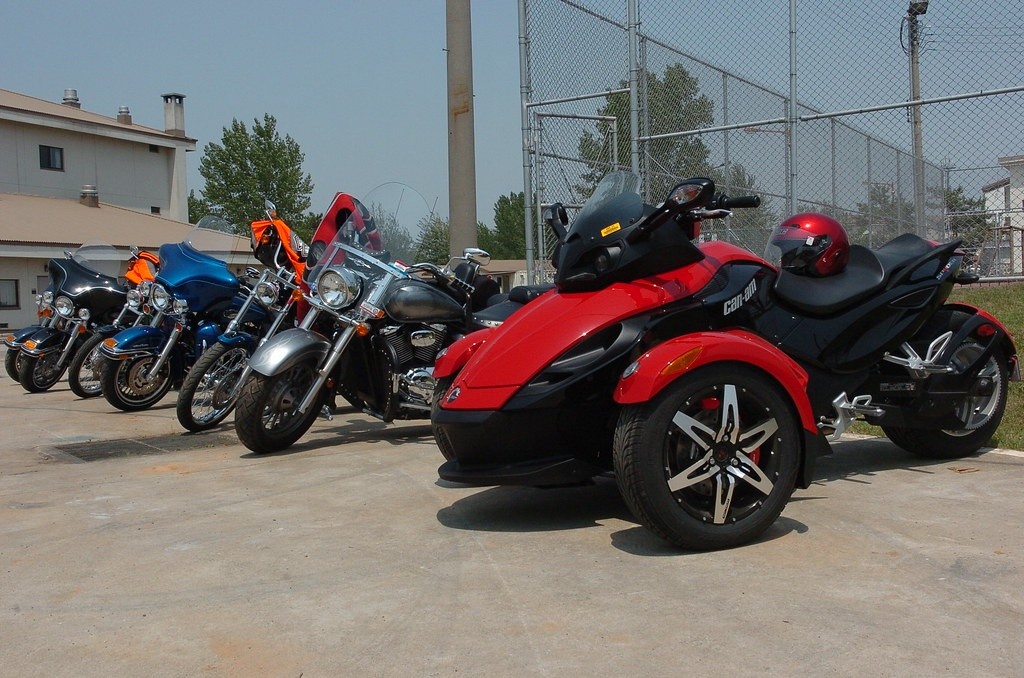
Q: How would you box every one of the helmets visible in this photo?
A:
[771,212,850,278]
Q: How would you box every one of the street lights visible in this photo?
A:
[898,0,930,237]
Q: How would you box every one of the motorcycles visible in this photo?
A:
[3,183,555,454]
[431,169,1019,554]
